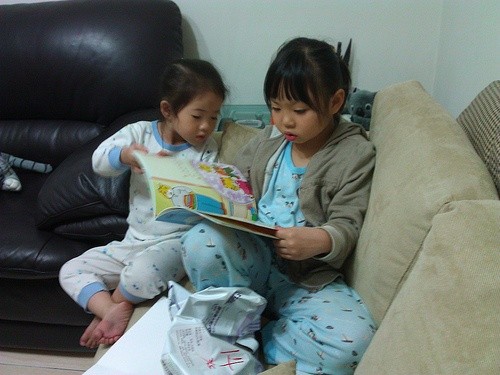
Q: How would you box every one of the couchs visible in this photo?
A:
[0,0,193,285]
[77,78,500,374]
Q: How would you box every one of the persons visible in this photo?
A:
[58,58,220,348]
[178,36,378,375]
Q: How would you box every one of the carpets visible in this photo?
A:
[0,279,97,356]
